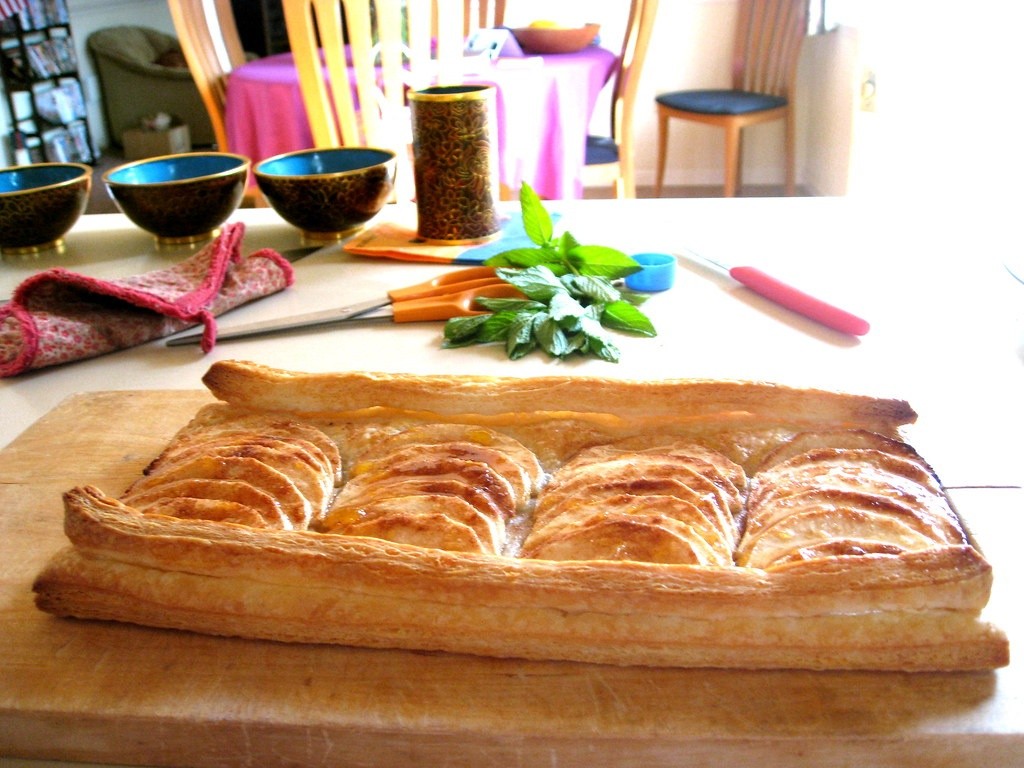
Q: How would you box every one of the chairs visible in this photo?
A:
[167,0,658,198]
[654,0,809,197]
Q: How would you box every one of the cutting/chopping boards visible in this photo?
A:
[0,387,1023,768]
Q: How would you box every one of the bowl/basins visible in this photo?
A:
[512,24,601,55]
[101,152,252,244]
[0,162,94,254]
[252,145,397,248]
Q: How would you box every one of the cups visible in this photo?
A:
[405,84,502,246]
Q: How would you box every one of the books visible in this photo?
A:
[0,0,93,165]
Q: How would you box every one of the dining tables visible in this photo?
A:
[0,198,1024,768]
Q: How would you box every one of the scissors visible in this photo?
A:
[164,265,534,346]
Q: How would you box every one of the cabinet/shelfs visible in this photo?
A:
[0,0,97,169]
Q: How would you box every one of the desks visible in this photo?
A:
[226,38,617,200]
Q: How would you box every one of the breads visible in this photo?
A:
[29,357,1009,674]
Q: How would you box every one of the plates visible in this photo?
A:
[343,212,562,267]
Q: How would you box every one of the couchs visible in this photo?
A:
[87,26,219,151]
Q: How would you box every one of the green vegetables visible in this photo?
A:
[441,182,658,363]
